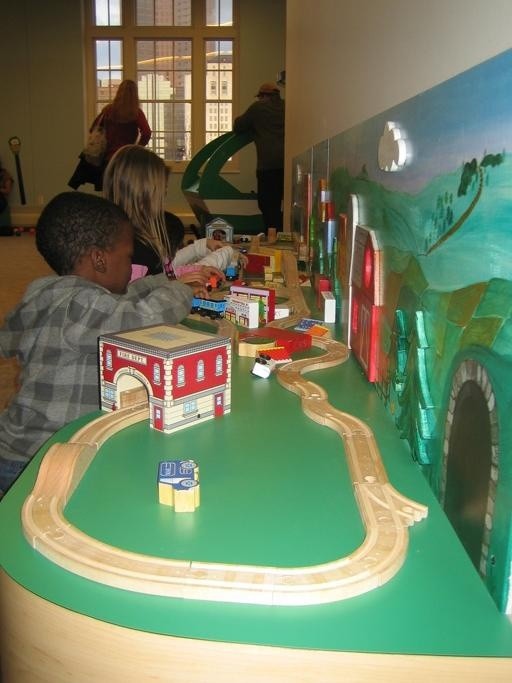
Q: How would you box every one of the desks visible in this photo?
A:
[1,232,509,677]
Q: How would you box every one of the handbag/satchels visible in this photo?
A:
[84,113,107,165]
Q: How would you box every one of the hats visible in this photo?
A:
[259,83,280,94]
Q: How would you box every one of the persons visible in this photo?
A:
[102,143,248,284]
[67,80,151,191]
[0,191,211,502]
[234,84,284,235]
